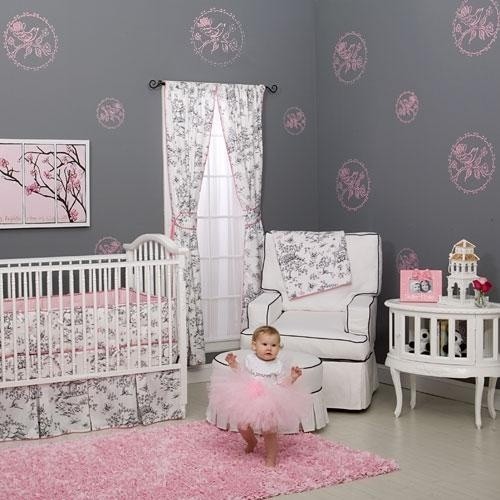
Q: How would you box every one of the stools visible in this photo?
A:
[204,349,330,435]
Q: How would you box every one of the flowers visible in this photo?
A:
[471,276,493,305]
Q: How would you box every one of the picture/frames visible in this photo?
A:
[0,137,92,229]
[400,269,443,304]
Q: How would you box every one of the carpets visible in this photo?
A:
[0,418,401,500]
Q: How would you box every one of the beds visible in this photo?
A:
[0,232,188,441]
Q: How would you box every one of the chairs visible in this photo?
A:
[240,230,384,414]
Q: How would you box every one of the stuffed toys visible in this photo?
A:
[443,330,466,357]
[409,328,430,355]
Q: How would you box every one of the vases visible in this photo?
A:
[473,293,490,308]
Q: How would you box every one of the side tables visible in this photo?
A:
[382,296,500,431]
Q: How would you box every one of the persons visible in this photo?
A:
[411,280,431,294]
[208,326,313,468]
